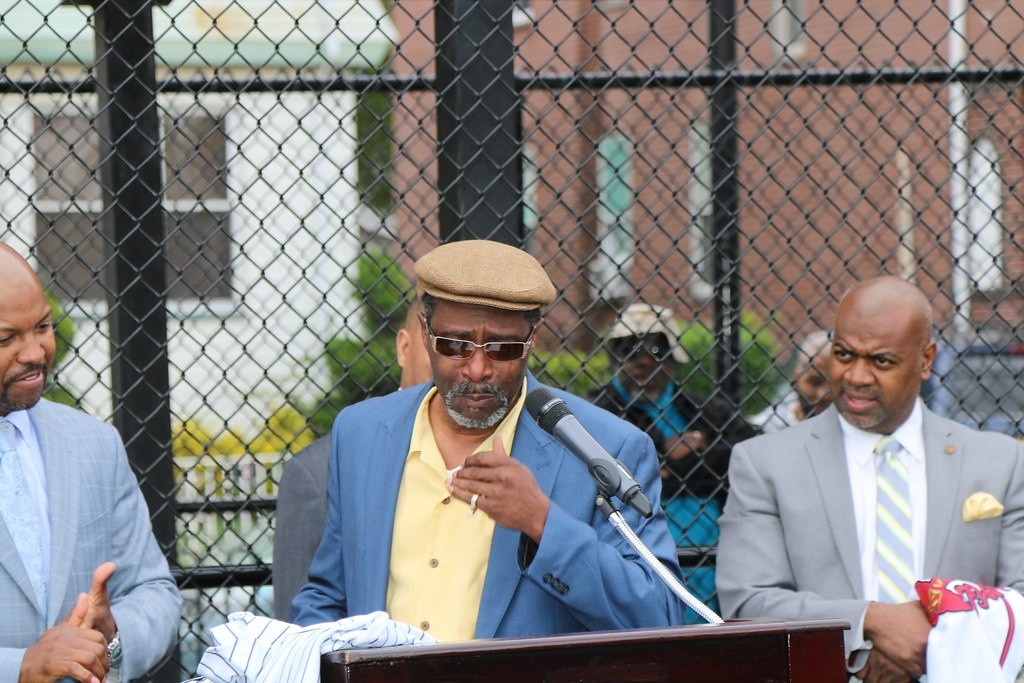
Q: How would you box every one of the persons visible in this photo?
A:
[290,240,687,642]
[273,295,432,624]
[586,304,764,625]
[714,276,1023,683]
[750,333,834,434]
[0,241,183,683]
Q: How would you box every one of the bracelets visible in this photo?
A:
[692,430,703,441]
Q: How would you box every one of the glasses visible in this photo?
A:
[423,316,536,361]
[621,344,667,360]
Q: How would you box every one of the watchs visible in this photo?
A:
[109,630,123,667]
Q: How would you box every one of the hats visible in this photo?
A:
[413,239,557,311]
[606,303,690,365]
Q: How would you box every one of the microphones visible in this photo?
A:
[525,387,653,519]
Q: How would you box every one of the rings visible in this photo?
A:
[469,493,480,514]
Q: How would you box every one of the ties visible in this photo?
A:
[873,435,914,607]
[0,422,47,617]
